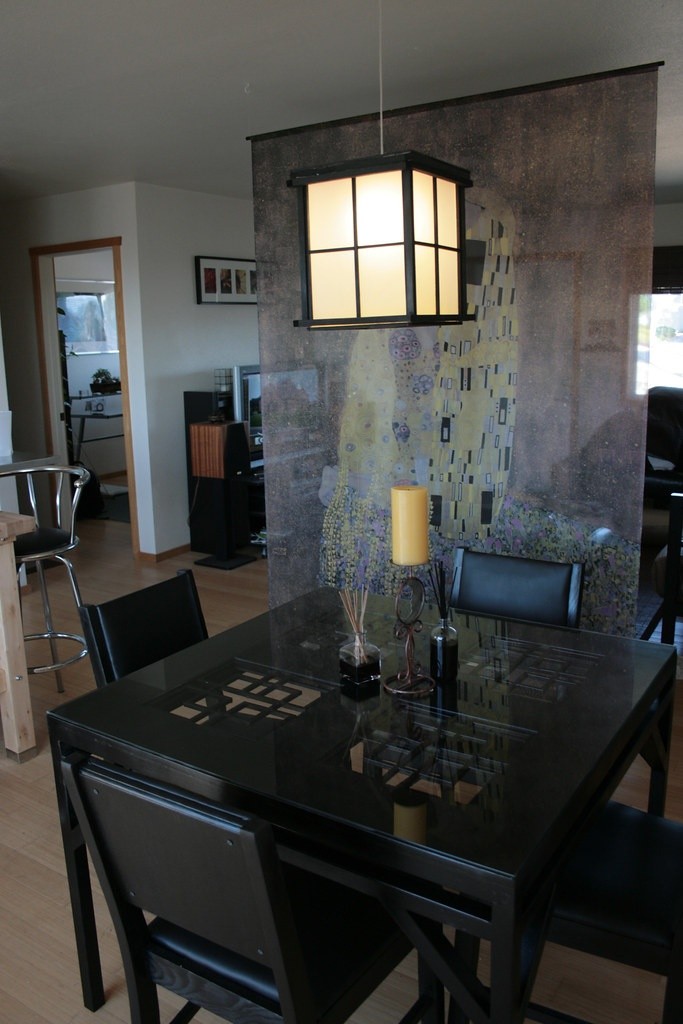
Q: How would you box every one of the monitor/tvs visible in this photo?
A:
[232,357,332,477]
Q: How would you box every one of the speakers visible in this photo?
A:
[190,421,252,479]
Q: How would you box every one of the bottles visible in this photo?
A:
[430,617,458,680]
[339,629,380,685]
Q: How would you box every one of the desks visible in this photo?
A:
[0,510,37,763]
[46,586,683,1024]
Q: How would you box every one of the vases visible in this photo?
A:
[335,630,380,685]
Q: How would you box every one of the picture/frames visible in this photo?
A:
[194,255,257,305]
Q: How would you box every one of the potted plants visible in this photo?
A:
[90,369,122,394]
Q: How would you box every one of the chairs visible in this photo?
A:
[449,543,583,631]
[77,567,210,684]
[61,752,446,1024]
[525,801,683,1024]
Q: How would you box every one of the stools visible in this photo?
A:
[0,467,91,693]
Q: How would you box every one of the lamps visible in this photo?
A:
[381,484,437,695]
[293,0,478,332]
